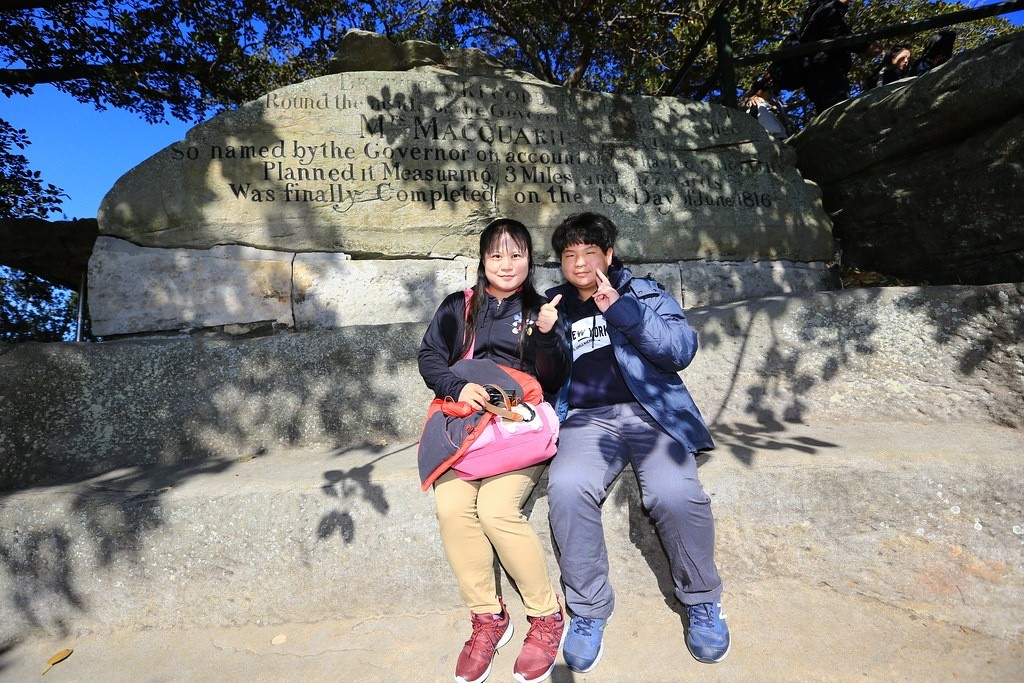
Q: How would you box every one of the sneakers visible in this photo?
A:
[454,595,515,683]
[562,609,614,672]
[512,593,566,683]
[685,594,732,664]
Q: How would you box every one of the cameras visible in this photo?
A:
[483,388,516,409]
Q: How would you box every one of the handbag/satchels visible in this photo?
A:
[450,383,561,482]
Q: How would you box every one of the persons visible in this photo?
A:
[418,218,565,683]
[868,40,911,91]
[909,30,956,78]
[800,0,881,116]
[747,78,789,142]
[545,211,731,673]
